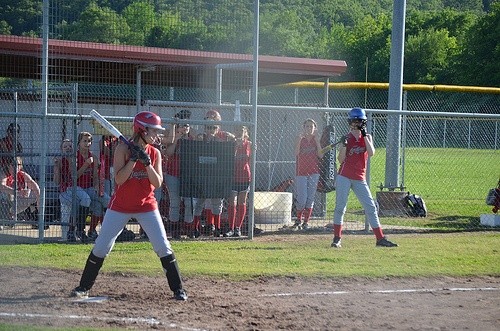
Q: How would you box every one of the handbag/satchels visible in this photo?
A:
[485,182,499,206]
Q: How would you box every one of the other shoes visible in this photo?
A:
[235,227,241,236]
[78,232,89,243]
[67,230,76,242]
[69,286,89,295]
[293,219,308,229]
[208,224,215,237]
[331,237,342,248]
[88,231,98,238]
[194,229,201,238]
[216,228,221,237]
[225,230,235,237]
[376,237,398,247]
[174,289,188,300]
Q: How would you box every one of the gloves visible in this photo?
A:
[128,144,141,162]
[137,147,151,164]
[338,135,348,147]
[356,125,366,137]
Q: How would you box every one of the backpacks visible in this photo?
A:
[404,191,426,217]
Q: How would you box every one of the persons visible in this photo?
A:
[69,110,187,301]
[54,110,255,241]
[0,122,23,171]
[0,154,48,230]
[293,118,323,229]
[331,107,399,248]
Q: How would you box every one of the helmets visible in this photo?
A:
[134,111,165,130]
[348,107,367,119]
[204,110,221,120]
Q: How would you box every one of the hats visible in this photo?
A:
[175,110,192,119]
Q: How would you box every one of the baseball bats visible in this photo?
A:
[89,108,149,165]
[319,134,352,154]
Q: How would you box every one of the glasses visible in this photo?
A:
[207,126,218,130]
[352,119,362,121]
